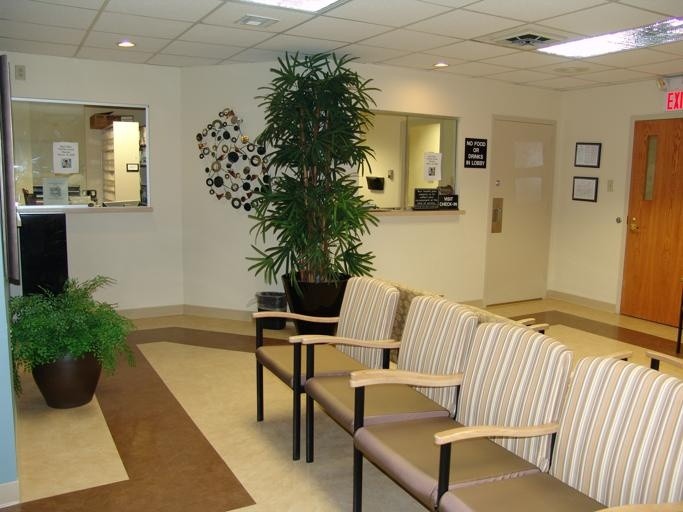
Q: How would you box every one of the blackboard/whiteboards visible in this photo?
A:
[413,188,440,210]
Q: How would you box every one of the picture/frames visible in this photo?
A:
[571,143,602,202]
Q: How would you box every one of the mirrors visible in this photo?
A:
[330,107,458,216]
[10,96,150,212]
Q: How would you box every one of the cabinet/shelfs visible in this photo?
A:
[103,122,145,202]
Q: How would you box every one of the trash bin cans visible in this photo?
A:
[255,292,287,330]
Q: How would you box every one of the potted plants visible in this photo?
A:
[245,47,382,338]
[10,274,139,410]
